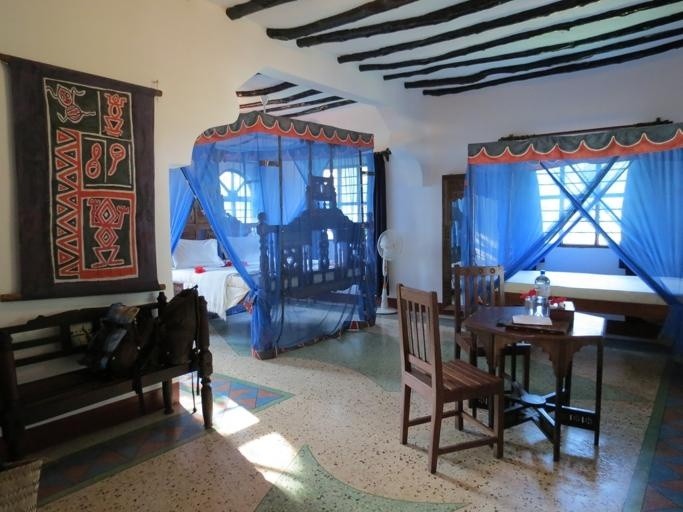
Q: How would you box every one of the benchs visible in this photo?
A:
[0,292,213,462]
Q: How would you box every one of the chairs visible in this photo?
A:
[395,283,505,474]
[452,264,532,411]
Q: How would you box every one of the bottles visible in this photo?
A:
[534,270,551,316]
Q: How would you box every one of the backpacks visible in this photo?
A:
[155,284,202,367]
[80,307,156,377]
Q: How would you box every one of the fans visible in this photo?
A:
[373,230,402,315]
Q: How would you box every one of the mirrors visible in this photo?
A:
[450,194,499,307]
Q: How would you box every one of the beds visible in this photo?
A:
[494,270,683,326]
[169,167,376,360]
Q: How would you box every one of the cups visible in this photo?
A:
[525,298,535,314]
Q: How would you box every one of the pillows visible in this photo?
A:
[224,235,261,260]
[172,238,226,270]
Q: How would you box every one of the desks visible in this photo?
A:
[464,305,607,463]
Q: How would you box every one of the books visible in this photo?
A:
[511,313,553,327]
[505,314,570,335]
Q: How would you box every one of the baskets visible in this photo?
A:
[0,455,46,512]
